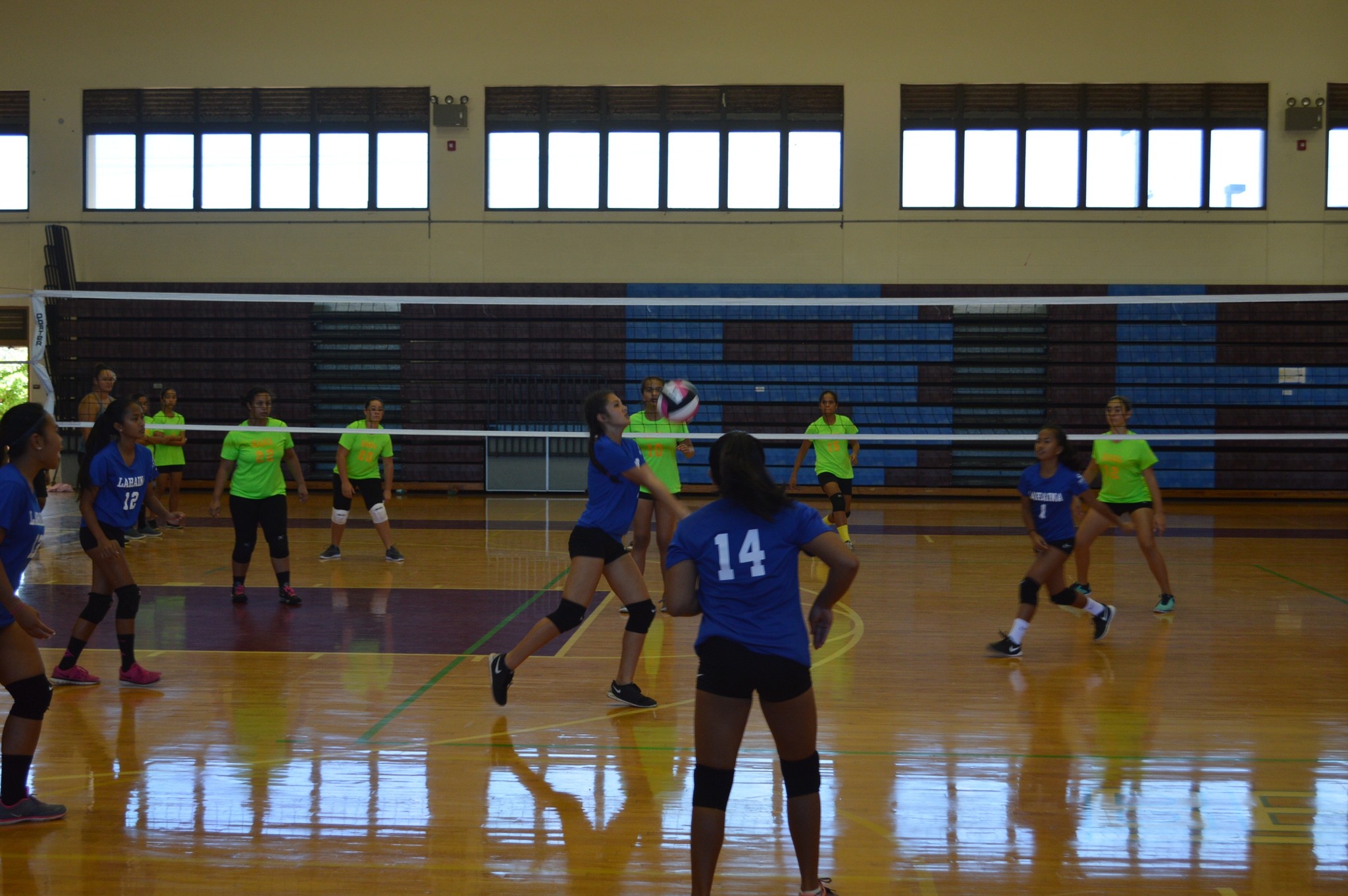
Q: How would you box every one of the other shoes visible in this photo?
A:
[798,880,840,896]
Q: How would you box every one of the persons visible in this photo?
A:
[318,396,405,562]
[489,389,695,709]
[1066,395,1177,615]
[71,364,188,546]
[619,376,693,616]
[211,387,309,608]
[984,424,1134,661]
[663,430,861,896]
[52,398,188,687]
[35,469,51,517]
[0,401,72,827]
[789,392,861,551]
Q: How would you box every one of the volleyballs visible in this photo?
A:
[657,378,700,423]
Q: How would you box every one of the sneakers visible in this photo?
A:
[985,630,1025,657]
[844,540,854,554]
[166,522,183,529]
[385,545,404,562]
[125,529,146,539]
[278,583,303,605]
[49,665,100,685]
[139,526,163,537]
[229,582,250,603]
[118,663,164,687]
[607,679,658,707]
[1091,603,1116,641]
[0,786,67,825]
[1153,594,1175,613]
[1067,582,1093,596]
[149,519,160,530]
[661,593,667,611]
[619,605,629,613]
[319,544,341,559]
[488,650,515,707]
